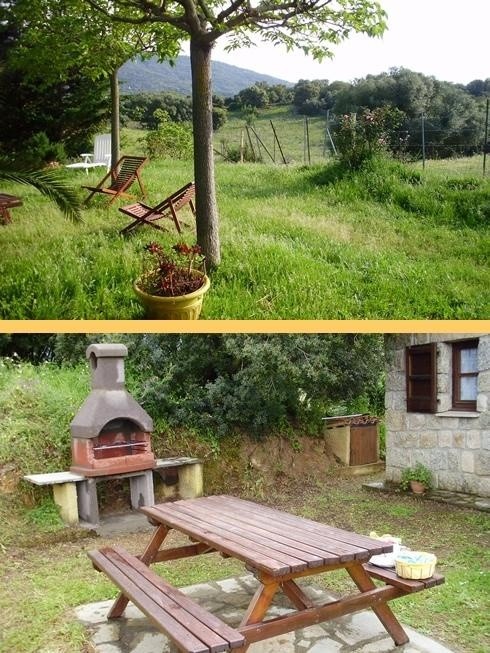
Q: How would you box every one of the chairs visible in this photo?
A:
[82,155,200,241]
[0,190,23,223]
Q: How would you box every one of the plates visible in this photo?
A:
[369,558,395,568]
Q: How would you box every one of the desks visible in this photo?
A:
[106,490,411,651]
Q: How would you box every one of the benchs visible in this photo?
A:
[356,554,446,647]
[83,542,247,653]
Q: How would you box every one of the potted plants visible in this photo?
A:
[400,461,432,494]
[133,239,211,320]
